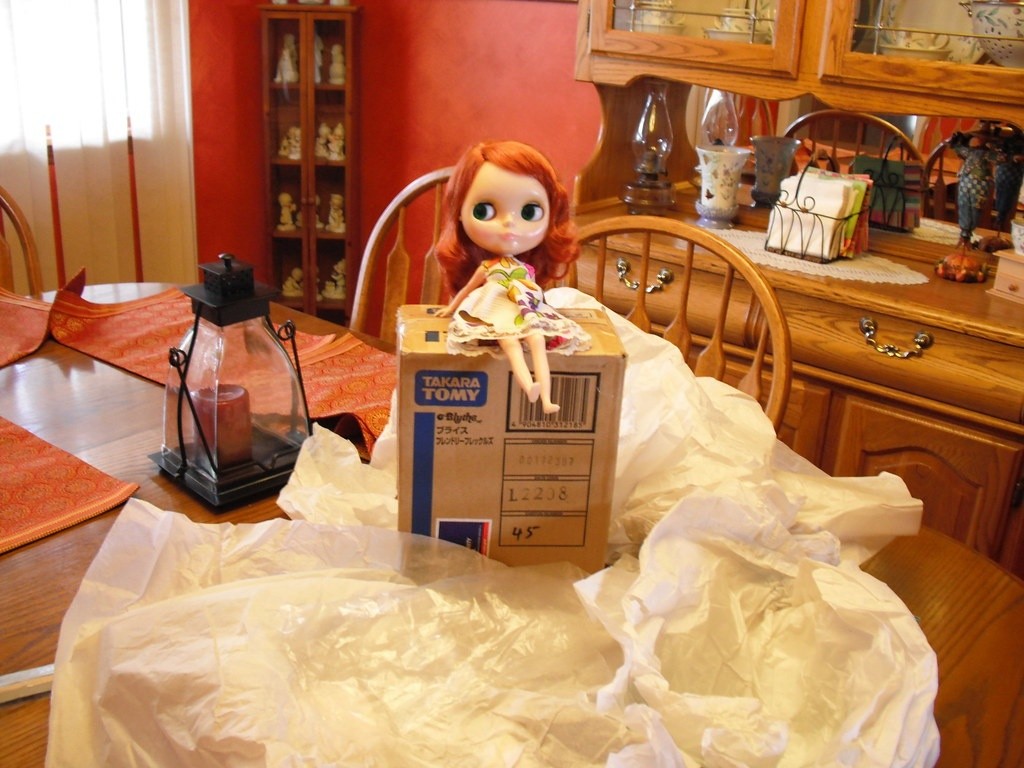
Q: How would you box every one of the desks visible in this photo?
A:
[0,282,1024,768]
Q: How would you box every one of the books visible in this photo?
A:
[764,166,873,261]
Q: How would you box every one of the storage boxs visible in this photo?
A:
[396,303,630,571]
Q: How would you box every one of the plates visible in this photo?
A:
[628,23,686,35]
[874,0,987,64]
[875,44,952,59]
[705,29,767,42]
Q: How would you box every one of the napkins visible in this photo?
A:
[0,266,397,554]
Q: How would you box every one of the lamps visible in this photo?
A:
[619,91,680,213]
[148,253,312,509]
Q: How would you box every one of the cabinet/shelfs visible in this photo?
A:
[569,0,1024,577]
[262,6,358,316]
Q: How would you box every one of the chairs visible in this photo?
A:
[543,216,794,437]
[350,168,579,336]
[785,111,1023,230]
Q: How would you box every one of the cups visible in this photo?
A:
[630,0,685,23]
[713,9,756,30]
[895,27,949,49]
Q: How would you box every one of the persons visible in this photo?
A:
[273,28,348,298]
[435,139,590,414]
